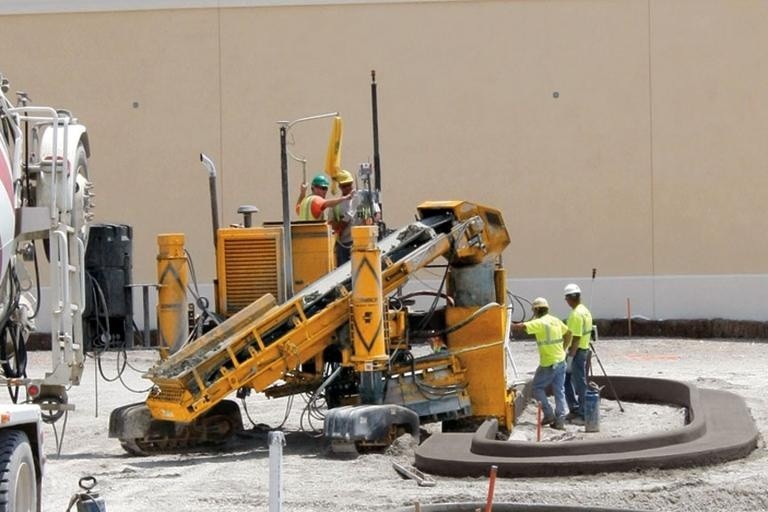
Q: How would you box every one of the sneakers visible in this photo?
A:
[542,413,586,430]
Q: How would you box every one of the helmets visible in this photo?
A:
[562,282,583,296]
[311,176,330,190]
[335,170,353,184]
[530,297,549,312]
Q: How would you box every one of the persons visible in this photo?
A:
[511,297,572,430]
[326,170,381,266]
[295,173,357,221]
[561,282,593,426]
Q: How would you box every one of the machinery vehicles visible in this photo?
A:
[1,78,110,511]
[108,68,522,456]
